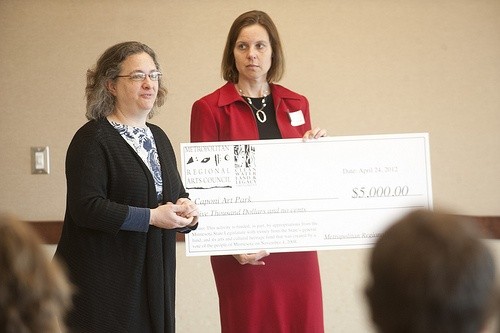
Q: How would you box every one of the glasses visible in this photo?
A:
[116,71,163,81]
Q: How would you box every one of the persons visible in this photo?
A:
[0,211,79,333]
[50,40,198,333]
[362,208,500,333]
[190,8,328,333]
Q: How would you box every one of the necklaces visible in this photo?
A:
[237,82,269,123]
